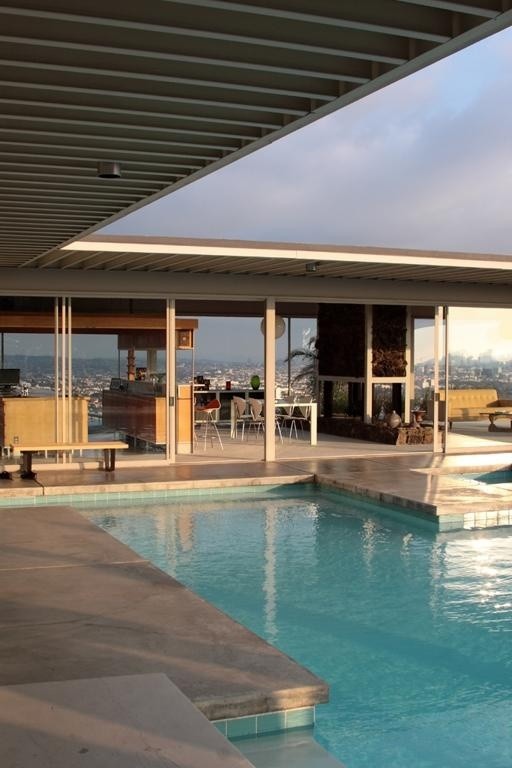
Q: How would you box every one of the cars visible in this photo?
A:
[226,380,231,390]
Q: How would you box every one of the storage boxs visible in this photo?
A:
[9,440,130,480]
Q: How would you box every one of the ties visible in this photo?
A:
[0,369,19,391]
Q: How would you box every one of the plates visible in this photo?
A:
[101,391,167,445]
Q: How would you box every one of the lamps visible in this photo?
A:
[230,395,318,446]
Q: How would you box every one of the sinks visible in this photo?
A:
[431,388,512,430]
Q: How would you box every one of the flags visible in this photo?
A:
[250,375,259,390]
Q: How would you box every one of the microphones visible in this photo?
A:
[196,399,224,452]
